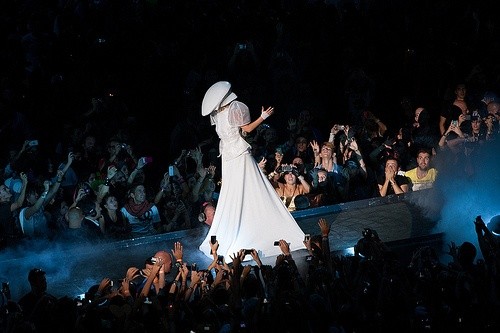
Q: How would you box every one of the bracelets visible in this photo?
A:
[323,236,328,238]
[160,188,166,192]
[113,152,116,156]
[391,182,396,185]
[355,149,359,152]
[136,168,141,171]
[41,191,48,198]
[442,132,449,137]
[172,280,178,283]
[306,249,311,252]
[198,180,203,185]
[74,201,77,204]
[188,287,194,289]
[21,150,22,152]
[56,180,62,183]
[211,284,215,287]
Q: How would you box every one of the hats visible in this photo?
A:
[201,81,238,117]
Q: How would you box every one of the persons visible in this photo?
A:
[199,80,307,264]
[0,83,500,333]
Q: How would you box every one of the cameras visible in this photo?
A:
[336,125,344,130]
[29,140,39,146]
[281,166,293,172]
[139,158,146,164]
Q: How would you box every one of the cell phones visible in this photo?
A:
[453,121,458,127]
[77,296,81,300]
[210,236,216,254]
[305,234,309,242]
[175,262,181,268]
[218,255,223,265]
[108,281,113,286]
[245,250,253,254]
[151,257,157,264]
[306,256,313,261]
[274,241,280,246]
[169,166,173,176]
